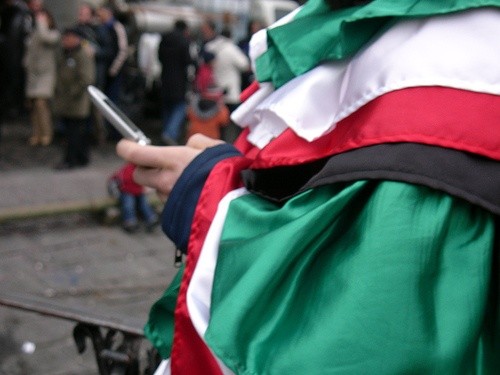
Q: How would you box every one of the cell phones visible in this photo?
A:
[87,84,152,172]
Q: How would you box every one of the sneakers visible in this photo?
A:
[125,219,138,231]
[55,162,74,168]
[146,217,160,228]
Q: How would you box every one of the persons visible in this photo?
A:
[183,84,231,148]
[193,18,217,76]
[93,5,129,143]
[237,18,262,88]
[73,3,113,149]
[116,0,499,375]
[18,0,55,59]
[155,18,198,146]
[51,28,97,170]
[199,28,252,141]
[193,50,216,92]
[106,161,161,230]
[104,0,140,124]
[20,8,61,148]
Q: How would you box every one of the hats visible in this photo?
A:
[63,29,88,39]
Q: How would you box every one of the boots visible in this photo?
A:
[41,118,53,146]
[27,118,40,145]
